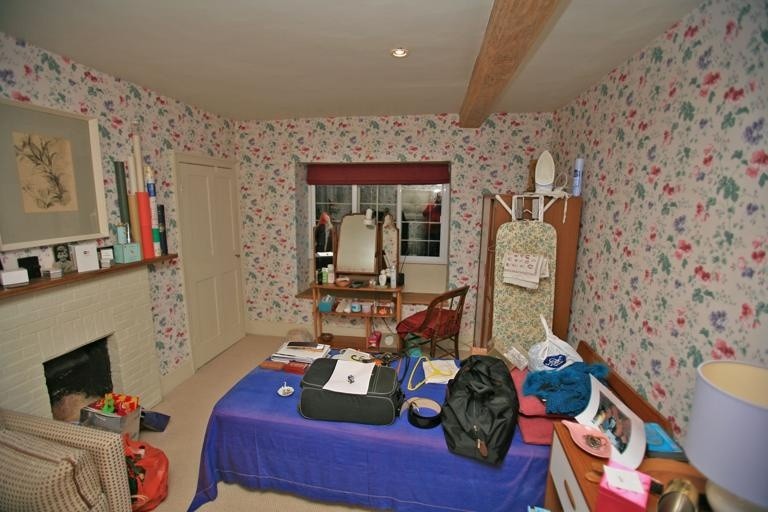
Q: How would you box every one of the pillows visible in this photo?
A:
[508,365,576,446]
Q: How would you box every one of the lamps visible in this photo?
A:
[684,359,768,511]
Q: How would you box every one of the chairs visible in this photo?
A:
[397,286,472,359]
[0,406,131,511]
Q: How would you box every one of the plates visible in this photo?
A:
[278,385,294,397]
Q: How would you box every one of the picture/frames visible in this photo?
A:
[0,97,110,253]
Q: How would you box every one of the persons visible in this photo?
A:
[421,192,442,257]
[590,401,632,453]
[55,245,72,272]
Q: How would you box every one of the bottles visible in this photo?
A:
[116,223,128,244]
[361,303,371,314]
[351,298,361,313]
[571,158,585,197]
[378,263,396,289]
[315,264,334,284]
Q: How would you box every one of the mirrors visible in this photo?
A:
[332,213,382,275]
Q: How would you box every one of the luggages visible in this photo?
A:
[300,357,404,425]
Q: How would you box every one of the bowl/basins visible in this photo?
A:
[336,277,350,287]
[319,332,334,342]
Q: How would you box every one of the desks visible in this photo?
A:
[309,278,404,350]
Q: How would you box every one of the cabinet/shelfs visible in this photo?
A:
[477,193,583,352]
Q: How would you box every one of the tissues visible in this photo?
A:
[380,332,399,352]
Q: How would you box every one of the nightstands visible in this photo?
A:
[546,420,710,512]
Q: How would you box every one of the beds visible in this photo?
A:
[195,335,672,512]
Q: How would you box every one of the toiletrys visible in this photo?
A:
[378,269,387,286]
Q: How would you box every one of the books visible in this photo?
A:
[255,340,331,375]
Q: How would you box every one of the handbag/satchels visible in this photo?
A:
[441,355,519,465]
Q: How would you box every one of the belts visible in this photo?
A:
[399,397,442,429]
